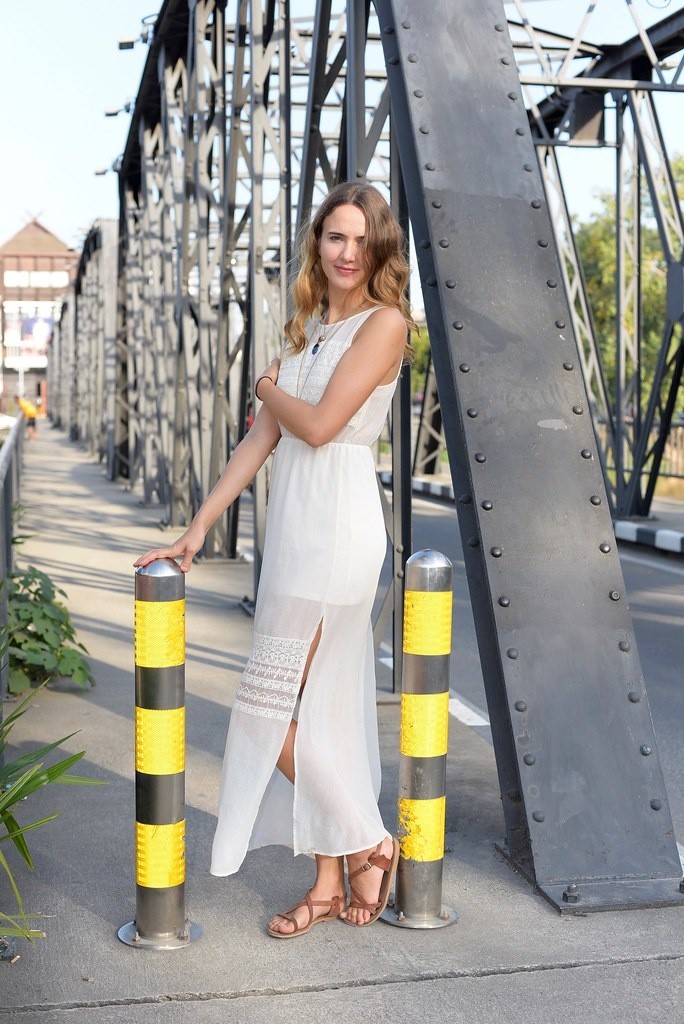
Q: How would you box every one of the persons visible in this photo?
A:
[36,396,42,414]
[132,183,422,939]
[14,395,38,442]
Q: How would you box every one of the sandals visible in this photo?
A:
[344,838,399,927]
[267,888,348,938]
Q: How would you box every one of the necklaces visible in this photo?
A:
[297,299,368,399]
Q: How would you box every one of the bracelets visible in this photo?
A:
[255,376,273,401]
